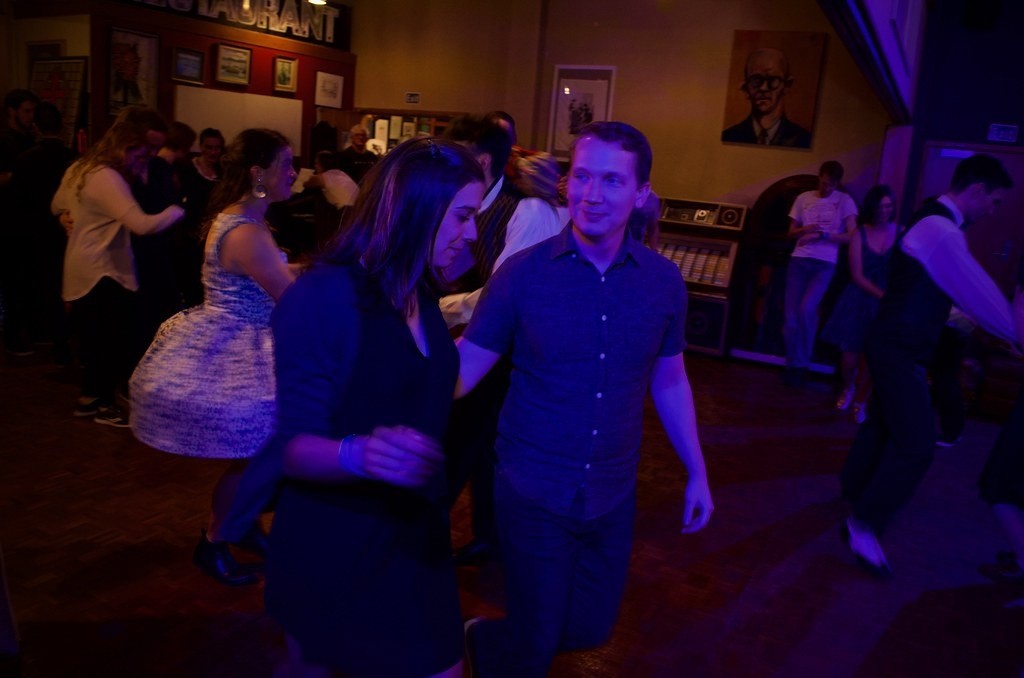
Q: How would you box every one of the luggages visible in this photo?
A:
[195,529,257,589]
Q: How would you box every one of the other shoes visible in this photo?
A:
[835,388,853,411]
[852,403,867,425]
[840,520,895,582]
[936,439,954,448]
[8,344,33,356]
[453,540,500,563]
[464,617,483,678]
[782,368,804,389]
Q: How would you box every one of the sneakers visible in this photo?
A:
[95,407,131,430]
[73,399,106,417]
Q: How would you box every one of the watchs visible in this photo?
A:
[816,230,823,242]
[338,433,359,476]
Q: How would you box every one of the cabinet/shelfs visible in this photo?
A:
[318,106,472,164]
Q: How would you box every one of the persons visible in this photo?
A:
[193,127,297,587]
[831,184,909,426]
[0,88,715,678]
[789,152,1024,578]
[721,48,811,148]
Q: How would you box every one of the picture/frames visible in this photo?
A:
[103,24,162,121]
[170,44,205,87]
[312,70,346,111]
[214,41,253,88]
[27,55,89,157]
[545,64,617,162]
[273,54,300,95]
